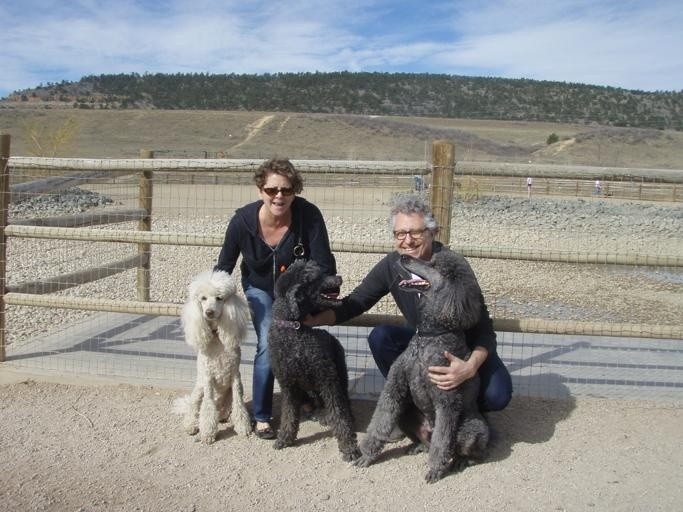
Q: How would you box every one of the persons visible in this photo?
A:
[594,181,602,194]
[526,176,534,196]
[304,199,513,443]
[213,158,337,439]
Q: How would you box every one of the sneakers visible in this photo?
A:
[387,424,405,443]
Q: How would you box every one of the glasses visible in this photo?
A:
[264,187,295,197]
[393,229,428,240]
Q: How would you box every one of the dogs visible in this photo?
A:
[266,257,363,463]
[352,246,492,485]
[179,267,253,446]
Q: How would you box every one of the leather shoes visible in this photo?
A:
[253,419,275,439]
